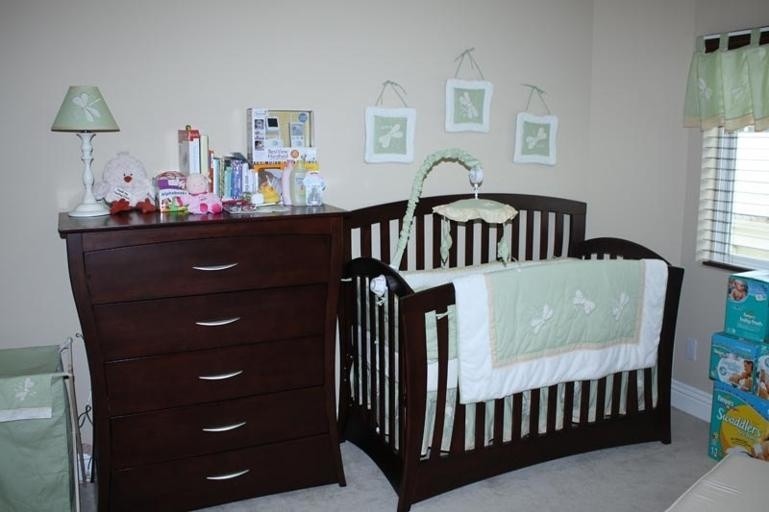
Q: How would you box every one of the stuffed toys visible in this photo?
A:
[95,154,158,214]
[176,174,223,214]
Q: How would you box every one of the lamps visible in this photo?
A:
[51,85,120,217]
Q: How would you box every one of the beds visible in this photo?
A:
[337,193,685,512]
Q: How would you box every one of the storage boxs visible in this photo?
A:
[707,267,769,461]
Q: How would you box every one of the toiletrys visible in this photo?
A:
[291,159,310,206]
[283,160,296,206]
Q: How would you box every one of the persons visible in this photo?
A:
[729,359,769,400]
[729,278,748,301]
[751,436,769,460]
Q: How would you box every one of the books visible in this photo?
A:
[179,126,259,203]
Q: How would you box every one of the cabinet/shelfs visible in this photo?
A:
[59,204,349,512]
